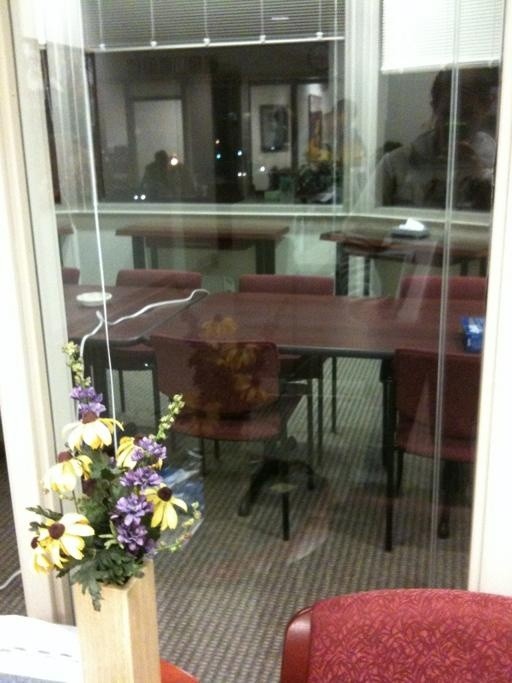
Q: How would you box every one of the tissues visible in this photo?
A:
[390,217,432,239]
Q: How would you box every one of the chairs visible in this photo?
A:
[278,587,512,683]
[233,273,342,457]
[64,267,205,412]
[379,275,487,550]
[140,339,312,546]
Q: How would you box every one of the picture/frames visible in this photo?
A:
[258,104,289,154]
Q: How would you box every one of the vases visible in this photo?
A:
[69,557,161,682]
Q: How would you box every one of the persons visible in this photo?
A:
[134,147,192,191]
[335,56,501,511]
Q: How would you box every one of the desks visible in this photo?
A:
[114,223,292,275]
[63,281,486,504]
[319,227,491,296]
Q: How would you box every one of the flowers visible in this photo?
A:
[23,340,207,613]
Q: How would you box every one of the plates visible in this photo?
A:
[76,291,112,308]
